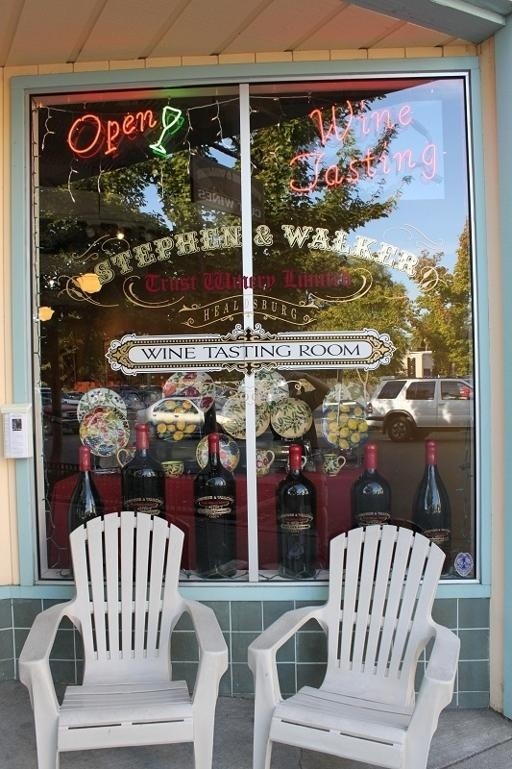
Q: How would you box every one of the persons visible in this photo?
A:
[269,372,330,449]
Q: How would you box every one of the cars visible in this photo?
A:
[41,376,289,439]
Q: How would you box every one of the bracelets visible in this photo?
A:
[304,373,308,379]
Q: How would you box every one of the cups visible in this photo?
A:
[256,448,274,478]
[161,460,185,478]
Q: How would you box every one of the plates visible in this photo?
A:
[148,369,373,450]
[76,386,131,459]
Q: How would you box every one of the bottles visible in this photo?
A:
[120,422,168,579]
[319,452,347,477]
[192,432,238,580]
[349,442,393,579]
[274,443,318,580]
[67,444,106,579]
[410,438,457,580]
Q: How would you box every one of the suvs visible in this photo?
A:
[365,374,479,444]
